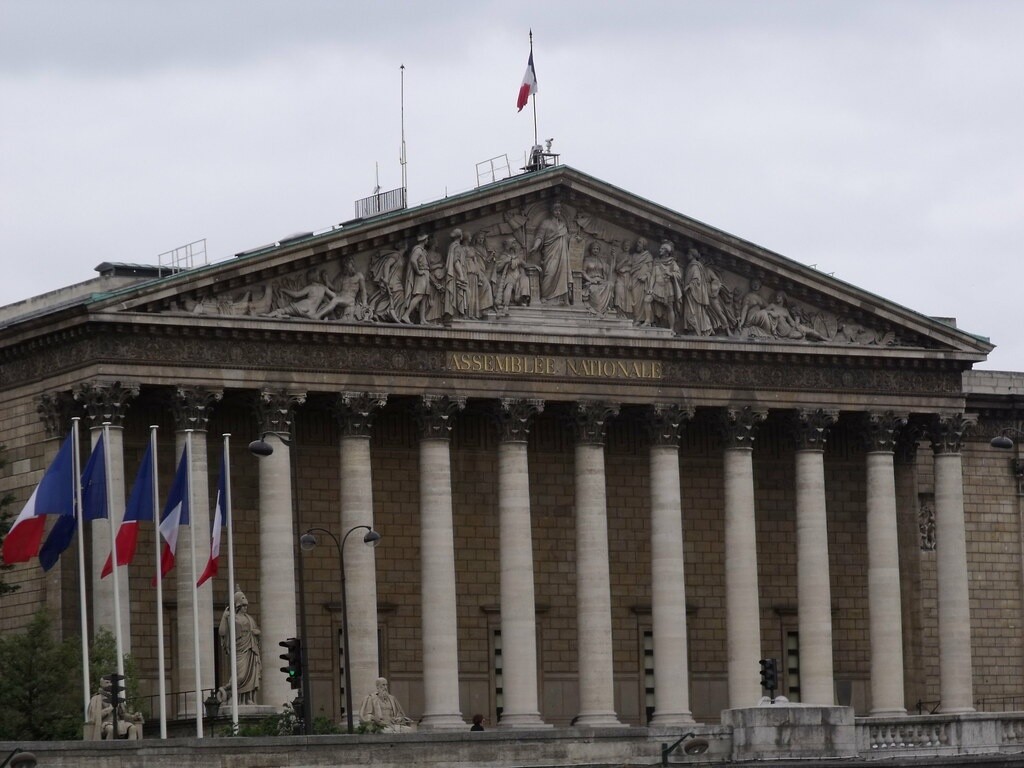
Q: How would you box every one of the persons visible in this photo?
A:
[367,234,446,325]
[258,268,337,318]
[444,229,496,320]
[359,677,414,733]
[471,714,484,731]
[305,254,368,320]
[737,279,832,342]
[86,678,143,740]
[491,238,530,316]
[582,236,735,337]
[530,201,574,306]
[218,592,264,704]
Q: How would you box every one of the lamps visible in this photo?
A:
[203,689,222,737]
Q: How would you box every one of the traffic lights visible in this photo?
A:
[278,637,302,690]
[759,657,778,691]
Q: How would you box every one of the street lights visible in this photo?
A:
[661,731,708,768]
[203,691,221,738]
[246,403,315,737]
[299,526,382,733]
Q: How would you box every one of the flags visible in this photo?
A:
[195,441,227,588]
[38,428,108,572]
[152,441,190,587]
[517,50,538,113]
[3,427,74,564]
[100,438,154,580]
